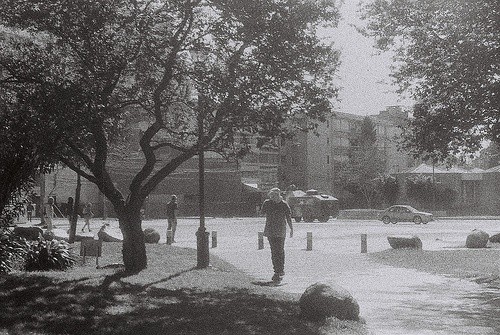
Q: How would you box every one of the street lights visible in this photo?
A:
[189,40,213,267]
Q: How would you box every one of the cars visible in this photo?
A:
[377,205,433,225]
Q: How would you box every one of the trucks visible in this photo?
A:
[289,196,339,223]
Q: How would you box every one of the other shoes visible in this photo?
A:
[272,272,285,285]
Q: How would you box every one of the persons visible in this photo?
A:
[27,197,95,234]
[165,195,178,244]
[259,188,293,286]
